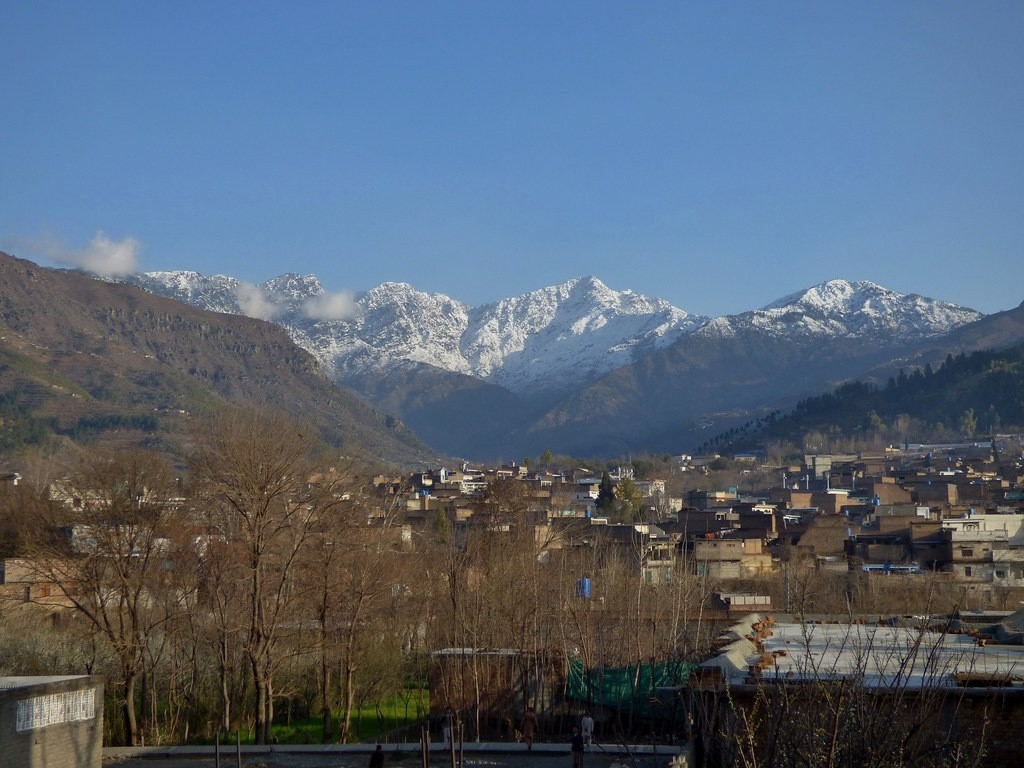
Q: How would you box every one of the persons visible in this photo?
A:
[440,706,457,751]
[581,710,595,748]
[570,725,585,768]
[520,706,540,752]
[683,713,695,742]
[368,745,384,768]
